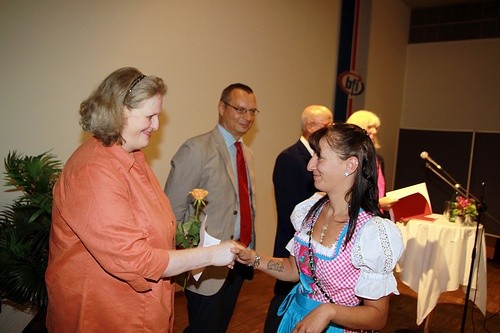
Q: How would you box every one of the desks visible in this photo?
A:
[391,214,489,333]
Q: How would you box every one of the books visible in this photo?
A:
[386,182,432,222]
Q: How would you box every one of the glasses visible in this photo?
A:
[225,102,260,116]
[313,122,335,129]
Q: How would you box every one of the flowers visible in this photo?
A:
[174,187,209,248]
[450,197,478,225]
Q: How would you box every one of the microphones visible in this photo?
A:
[420,151,443,170]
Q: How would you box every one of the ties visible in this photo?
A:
[235,141,253,248]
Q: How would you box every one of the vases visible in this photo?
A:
[456,211,467,222]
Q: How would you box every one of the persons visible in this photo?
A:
[263,105,336,333]
[228,123,405,333]
[44,67,238,333]
[164,81,261,332]
[347,111,387,217]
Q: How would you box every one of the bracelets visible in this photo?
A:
[249,251,262,269]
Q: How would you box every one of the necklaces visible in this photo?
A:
[319,202,349,244]
